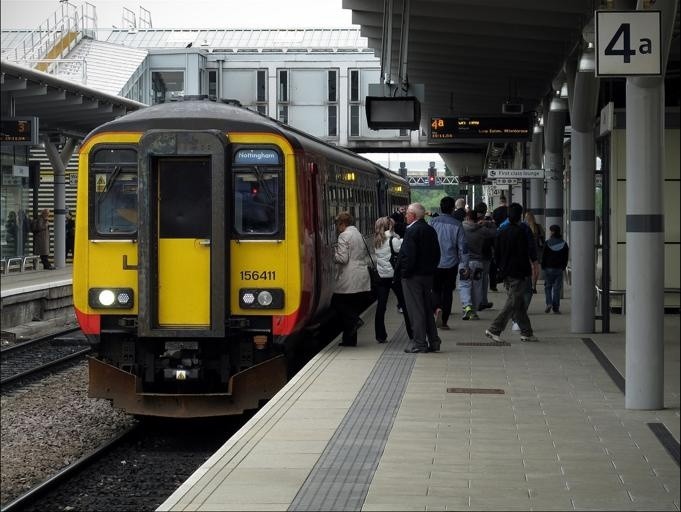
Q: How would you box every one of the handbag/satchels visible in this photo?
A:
[368,267,383,288]
[389,252,399,270]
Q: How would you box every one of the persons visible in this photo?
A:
[64,212,76,260]
[329,210,372,347]
[5,208,29,265]
[373,217,414,343]
[397,202,444,355]
[389,196,569,331]
[486,202,540,344]
[32,207,58,270]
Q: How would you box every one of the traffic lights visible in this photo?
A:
[398,162,408,179]
[427,162,438,188]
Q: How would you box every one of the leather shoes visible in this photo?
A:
[403,341,440,354]
[338,338,357,347]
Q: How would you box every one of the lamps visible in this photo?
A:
[531,80,568,134]
[577,40,596,73]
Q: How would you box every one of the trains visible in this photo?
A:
[72,94,412,418]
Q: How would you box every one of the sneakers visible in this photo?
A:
[545,304,562,314]
[461,302,493,320]
[434,308,449,330]
[485,322,538,343]
